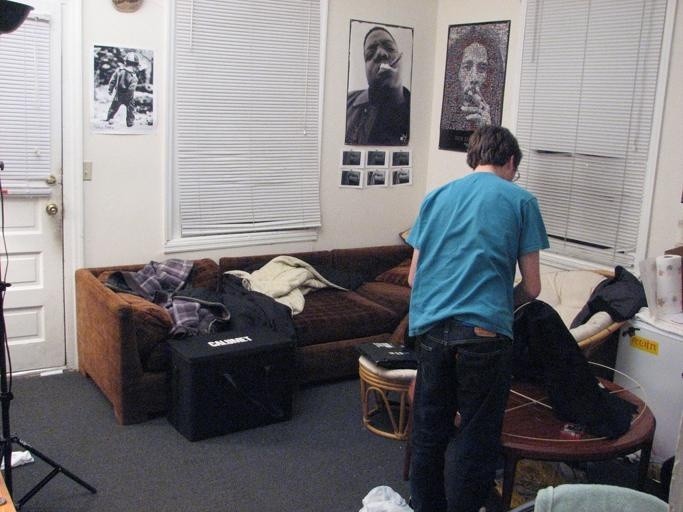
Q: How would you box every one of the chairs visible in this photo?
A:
[508,483,668,512]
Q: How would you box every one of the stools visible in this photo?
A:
[358,356,417,440]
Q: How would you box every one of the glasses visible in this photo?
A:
[513,164,520,182]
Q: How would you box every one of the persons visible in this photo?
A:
[405,125,550,512]
[98,50,140,129]
[441,27,504,133]
[343,27,408,147]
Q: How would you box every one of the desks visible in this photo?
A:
[405,362,656,512]
[164,326,291,442]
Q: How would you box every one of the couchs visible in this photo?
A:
[75,245,412,424]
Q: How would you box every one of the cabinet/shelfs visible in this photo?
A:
[613,316,683,466]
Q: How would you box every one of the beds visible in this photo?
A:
[506,269,624,358]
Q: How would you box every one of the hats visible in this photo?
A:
[123,52,140,63]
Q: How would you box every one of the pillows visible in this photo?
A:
[97,270,173,359]
[375,264,411,287]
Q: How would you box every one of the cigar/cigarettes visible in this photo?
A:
[472,92,482,102]
[388,52,402,68]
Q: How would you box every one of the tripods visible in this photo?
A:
[0,277,96,512]
